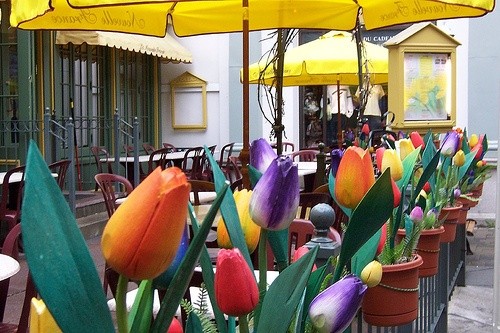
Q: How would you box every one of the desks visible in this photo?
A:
[114,191,216,205]
[225,143,251,152]
[107,265,279,321]
[118,152,205,168]
[0,254,20,282]
[293,162,330,188]
[0,173,59,186]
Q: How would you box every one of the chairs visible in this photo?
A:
[0,142,342,333]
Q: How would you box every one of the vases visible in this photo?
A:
[362,253,424,327]
[465,188,480,207]
[438,203,463,243]
[477,183,483,196]
[395,226,446,278]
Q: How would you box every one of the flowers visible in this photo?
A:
[22,123,497,333]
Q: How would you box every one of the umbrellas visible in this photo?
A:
[10,0,495,195]
[240,29,390,154]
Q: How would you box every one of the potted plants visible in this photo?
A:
[456,193,473,224]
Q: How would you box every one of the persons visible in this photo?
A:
[320,84,354,144]
[353,85,385,128]
